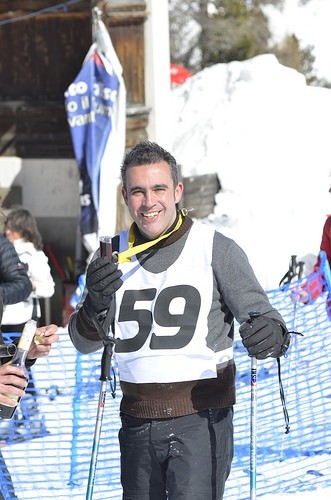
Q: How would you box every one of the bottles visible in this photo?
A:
[0,319,38,420]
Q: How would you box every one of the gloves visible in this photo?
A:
[86,257,124,313]
[239,316,285,359]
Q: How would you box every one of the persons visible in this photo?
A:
[0,207,59,500]
[290,215,331,320]
[69,141,290,500]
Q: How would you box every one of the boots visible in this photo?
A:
[0,394,44,437]
